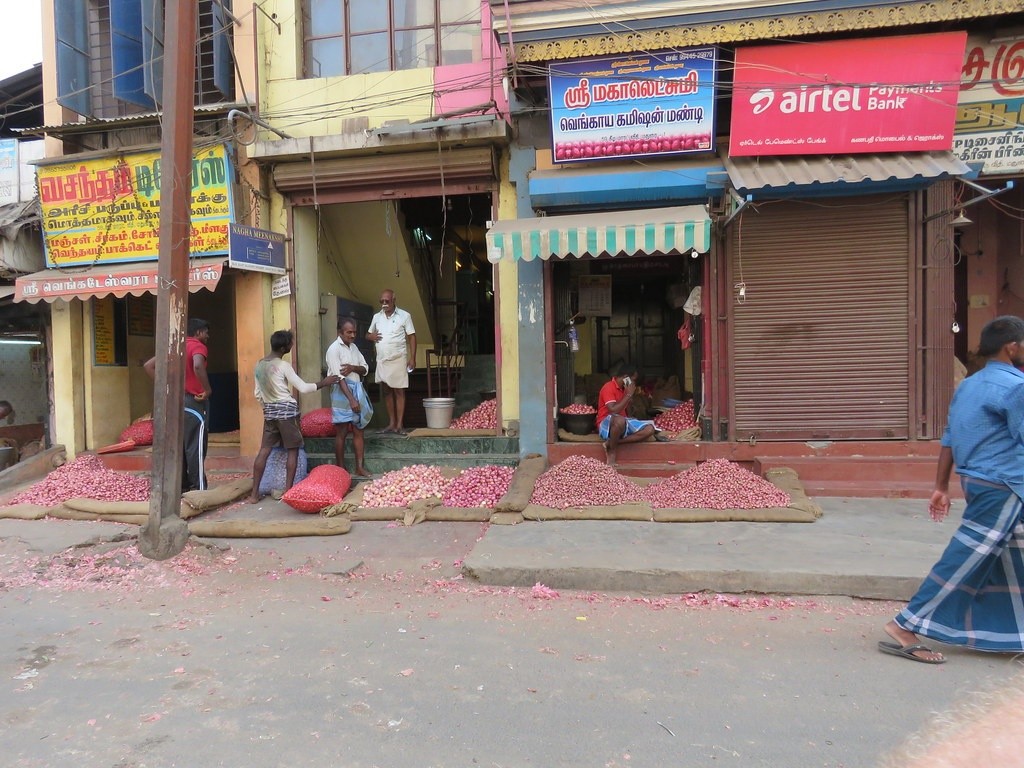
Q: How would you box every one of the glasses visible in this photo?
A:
[379,299,389,304]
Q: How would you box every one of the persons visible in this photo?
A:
[250,328,341,504]
[326,318,374,478]
[143,319,211,493]
[884,315,1024,662]
[365,289,417,434]
[596,364,655,467]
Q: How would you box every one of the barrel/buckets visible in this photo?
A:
[423,398,456,429]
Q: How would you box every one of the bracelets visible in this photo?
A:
[625,394,632,398]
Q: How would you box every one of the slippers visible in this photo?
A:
[878,641,948,664]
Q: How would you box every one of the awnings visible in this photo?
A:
[486,204,712,264]
[13,255,229,304]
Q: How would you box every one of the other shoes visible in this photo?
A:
[355,470,372,478]
[375,428,396,434]
[397,428,408,435]
[249,494,265,504]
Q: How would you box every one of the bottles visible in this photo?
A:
[569,319,579,352]
